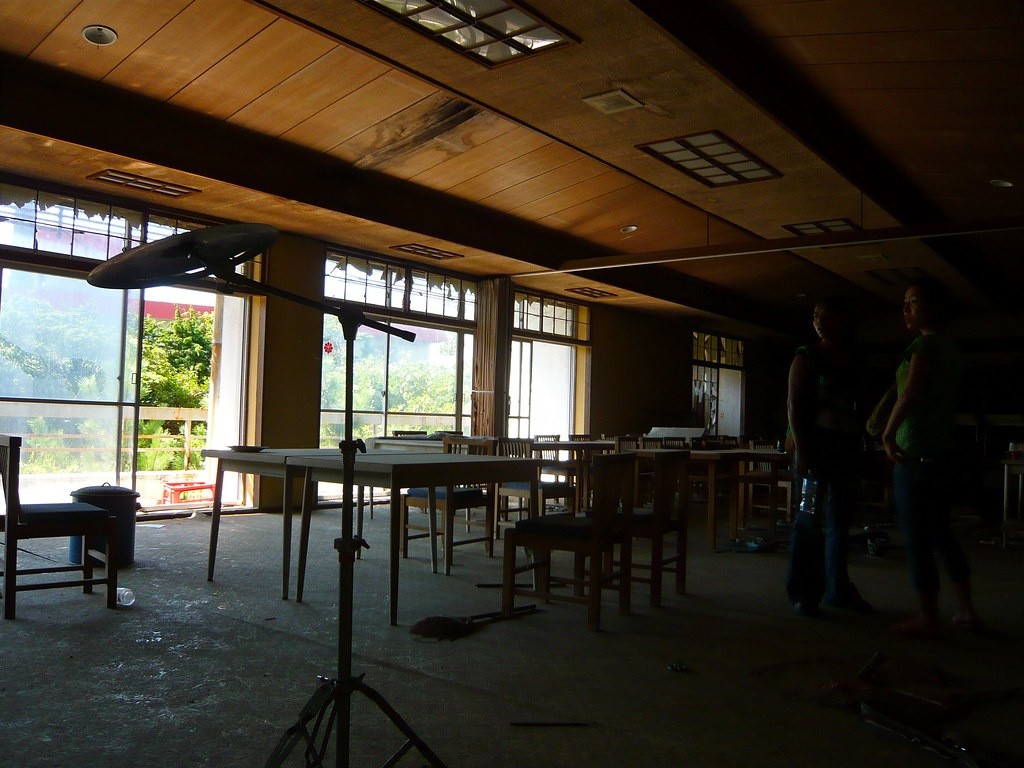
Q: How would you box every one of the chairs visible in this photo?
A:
[395,431,792,632]
[0,435,117,620]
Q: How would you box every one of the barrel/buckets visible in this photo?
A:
[69,483,141,571]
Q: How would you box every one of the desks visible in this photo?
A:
[533,442,615,512]
[202,449,554,625]
[626,449,786,549]
[1001,459,1024,561]
[365,437,523,533]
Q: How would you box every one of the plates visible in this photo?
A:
[227,445,268,453]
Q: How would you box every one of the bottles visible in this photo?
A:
[800,470,819,513]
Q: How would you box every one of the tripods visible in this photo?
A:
[200,257,446,768]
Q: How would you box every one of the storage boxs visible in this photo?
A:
[162,482,215,505]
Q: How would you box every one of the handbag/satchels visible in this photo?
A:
[866,381,898,437]
[784,345,817,453]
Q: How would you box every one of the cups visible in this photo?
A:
[117,587,135,607]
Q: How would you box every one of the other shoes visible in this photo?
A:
[823,597,870,609]
[888,617,941,637]
[792,601,816,615]
[941,618,985,634]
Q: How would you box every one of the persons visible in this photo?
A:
[783,294,874,618]
[881,277,985,637]
